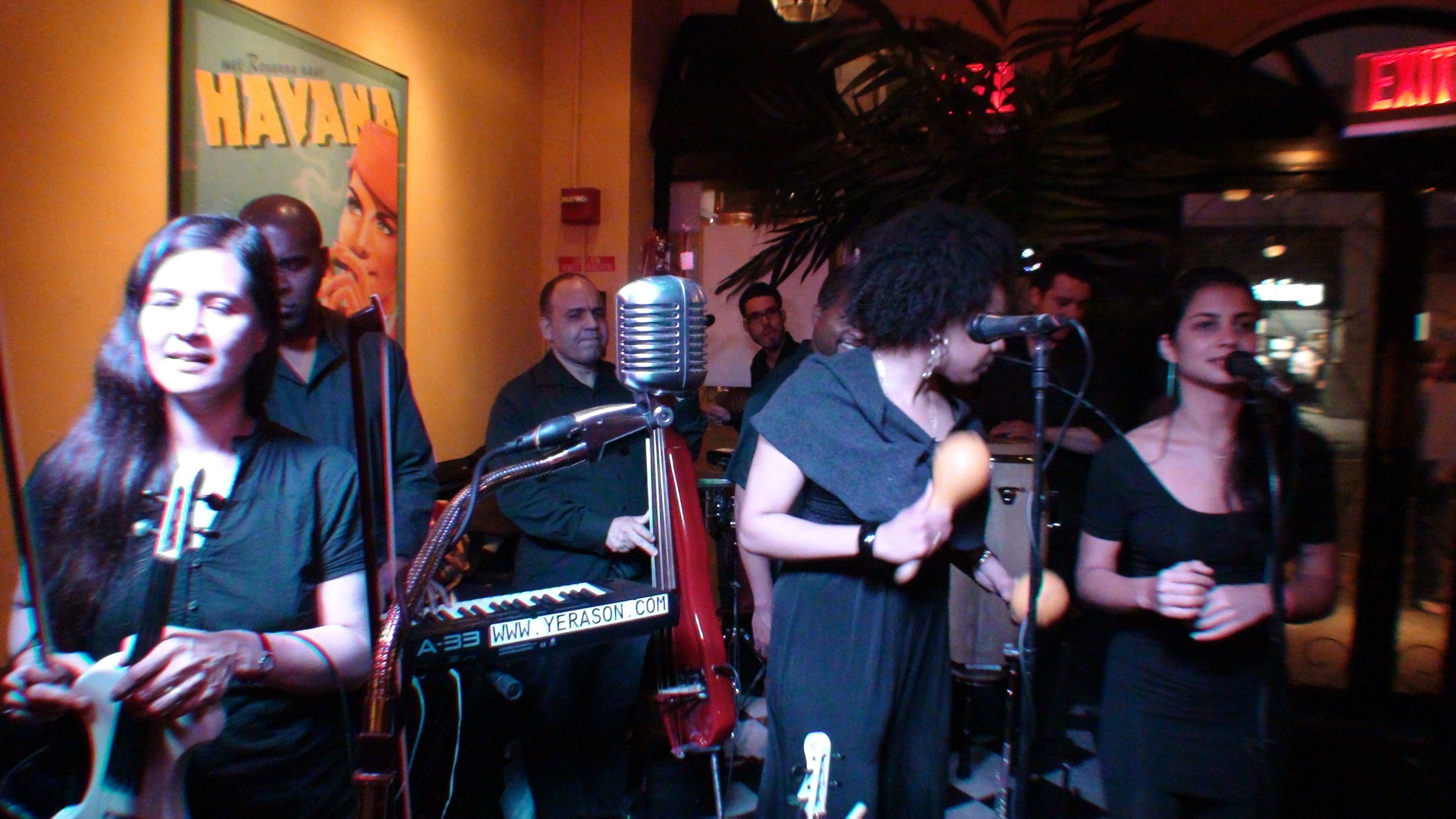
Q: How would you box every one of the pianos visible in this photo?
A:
[376,575,682,678]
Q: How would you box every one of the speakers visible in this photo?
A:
[944,452,1051,673]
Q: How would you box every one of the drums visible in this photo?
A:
[946,433,1050,687]
[694,476,742,536]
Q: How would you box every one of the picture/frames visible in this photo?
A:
[166,0,411,348]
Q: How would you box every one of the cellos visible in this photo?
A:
[643,228,737,819]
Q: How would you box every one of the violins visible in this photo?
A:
[39,461,243,819]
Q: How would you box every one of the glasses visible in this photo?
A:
[747,306,781,324]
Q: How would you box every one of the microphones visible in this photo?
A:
[1224,350,1285,389]
[613,275,709,412]
[965,311,1070,344]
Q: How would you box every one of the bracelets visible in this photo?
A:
[858,520,880,565]
[1271,579,1287,621]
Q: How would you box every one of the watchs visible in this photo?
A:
[259,633,277,676]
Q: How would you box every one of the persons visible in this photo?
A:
[238,195,440,626]
[488,275,657,819]
[736,207,1068,818]
[738,282,802,395]
[1,215,371,819]
[941,245,1104,561]
[722,266,866,660]
[1075,267,1339,819]
